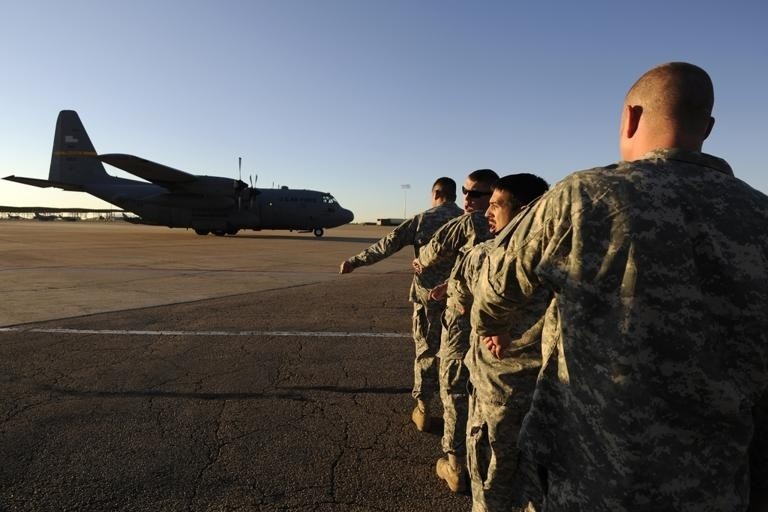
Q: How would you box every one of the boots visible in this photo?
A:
[436,453,467,492]
[412,398,432,432]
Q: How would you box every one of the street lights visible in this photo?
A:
[400,184,410,223]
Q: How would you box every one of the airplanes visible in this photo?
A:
[7,212,81,221]
[122,214,142,224]
[1,110,353,238]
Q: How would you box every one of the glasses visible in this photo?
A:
[462,186,492,198]
